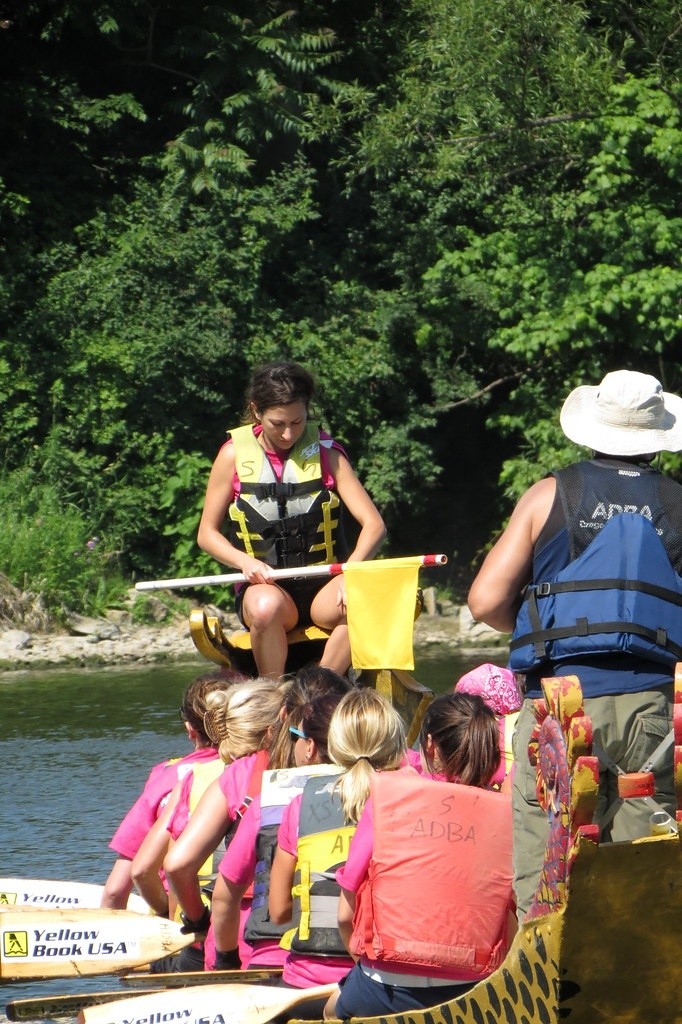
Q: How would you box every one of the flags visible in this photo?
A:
[345,561,418,669]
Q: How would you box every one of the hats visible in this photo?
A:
[560,370,682,456]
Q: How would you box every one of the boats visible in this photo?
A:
[0,610,682,1023]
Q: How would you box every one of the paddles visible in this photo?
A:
[0,876,158,921]
[0,908,205,980]
[77,980,340,1024]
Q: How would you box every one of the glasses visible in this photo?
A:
[288,726,309,743]
[178,706,189,721]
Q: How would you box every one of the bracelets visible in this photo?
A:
[180,911,209,934]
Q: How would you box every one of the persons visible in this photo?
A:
[466,370,682,930]
[196,362,387,677]
[102,665,513,1020]
